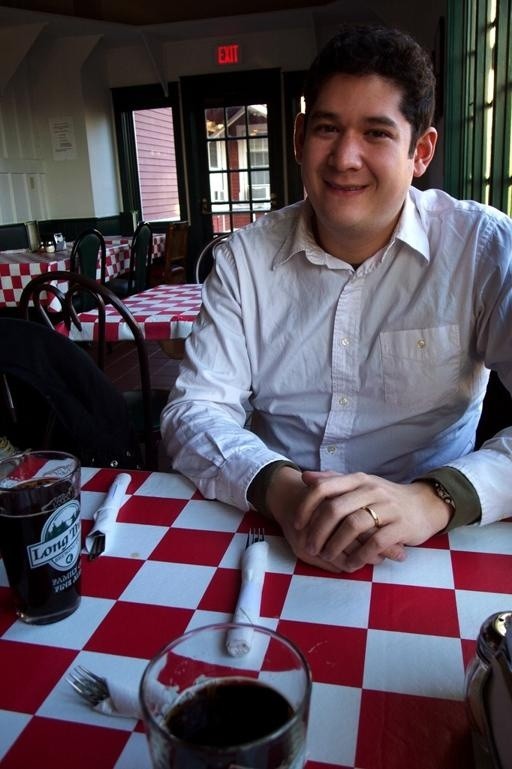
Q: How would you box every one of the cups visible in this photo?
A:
[138,622,312,769]
[0,450,82,625]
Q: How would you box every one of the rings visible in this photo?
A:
[362,506,378,527]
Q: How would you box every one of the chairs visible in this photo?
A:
[195,233,231,284]
[149,221,189,285]
[17,272,176,470]
[105,221,154,302]
[66,229,106,314]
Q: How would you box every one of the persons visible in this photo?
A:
[159,23,512,574]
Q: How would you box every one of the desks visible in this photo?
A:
[0,455,512,769]
[0,232,166,312]
[55,283,205,392]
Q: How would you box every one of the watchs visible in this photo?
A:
[431,478,455,508]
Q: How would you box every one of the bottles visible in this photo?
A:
[40,242,45,249]
[45,241,56,252]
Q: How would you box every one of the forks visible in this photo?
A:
[245,526,265,549]
[88,535,105,560]
[64,663,110,704]
